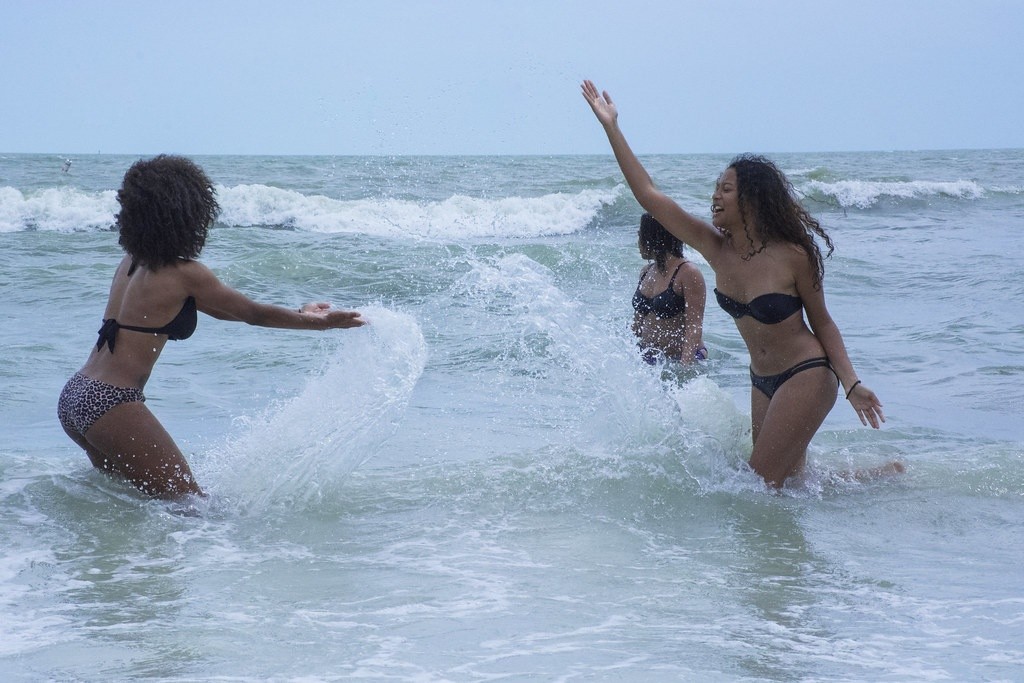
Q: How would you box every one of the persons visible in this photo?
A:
[632,213,708,364]
[58,154,364,499]
[579,78,884,491]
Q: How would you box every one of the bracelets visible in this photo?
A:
[846,380,861,398]
